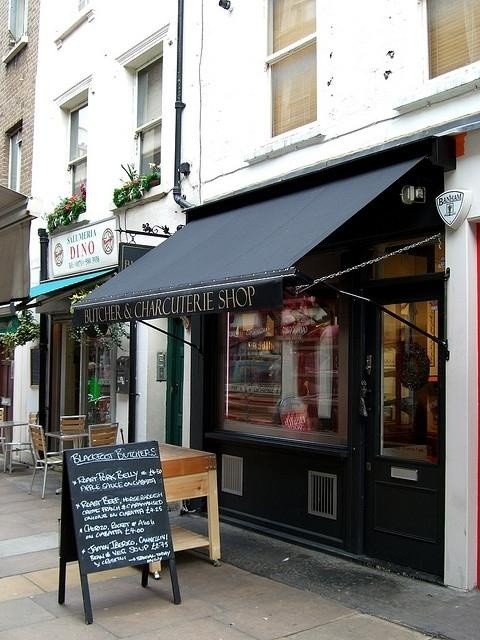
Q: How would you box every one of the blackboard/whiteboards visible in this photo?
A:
[65,441,175,574]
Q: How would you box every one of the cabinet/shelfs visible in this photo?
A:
[148,442,222,580]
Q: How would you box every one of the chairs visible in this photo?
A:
[0,407,119,500]
